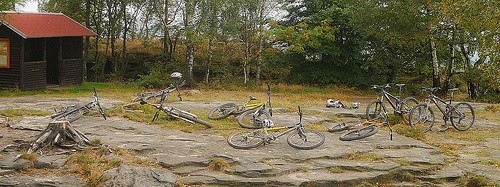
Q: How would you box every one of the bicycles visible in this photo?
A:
[329,117,393,141]
[206,83,274,123]
[133,72,188,104]
[228,105,325,150]
[49,86,107,125]
[409,82,474,136]
[367,83,422,125]
[139,90,211,129]
